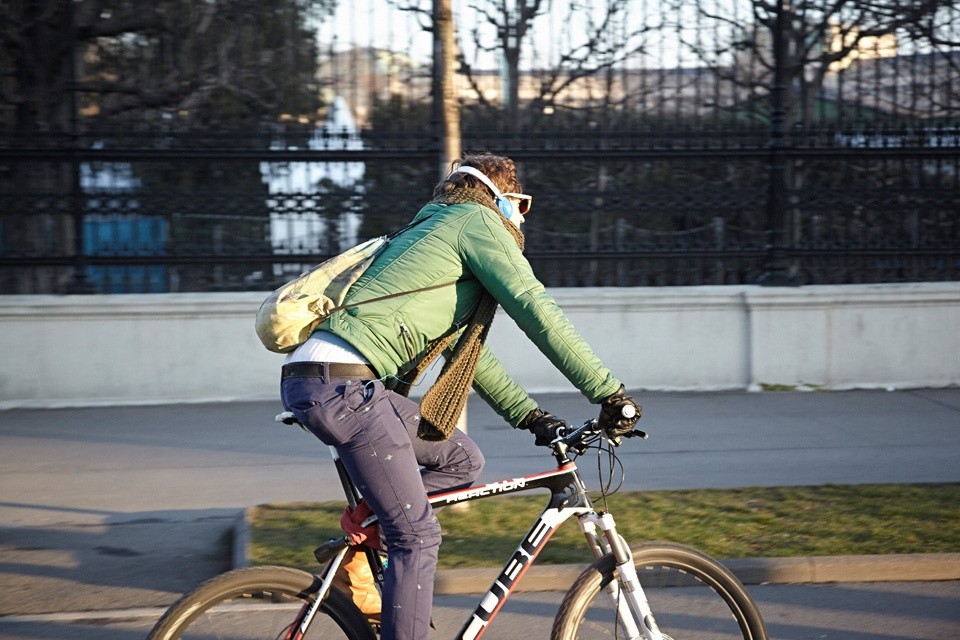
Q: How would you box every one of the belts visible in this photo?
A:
[282,362,380,379]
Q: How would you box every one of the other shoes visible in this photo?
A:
[320,538,382,622]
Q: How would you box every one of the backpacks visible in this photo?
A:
[255,215,476,353]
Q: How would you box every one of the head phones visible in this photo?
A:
[447,166,514,221]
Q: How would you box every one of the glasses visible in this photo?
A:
[493,193,532,215]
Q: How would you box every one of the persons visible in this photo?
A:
[279,151,639,640]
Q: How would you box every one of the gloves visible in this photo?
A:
[519,407,567,446]
[598,382,642,438]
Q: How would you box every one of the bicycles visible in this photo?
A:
[143,400,769,640]
